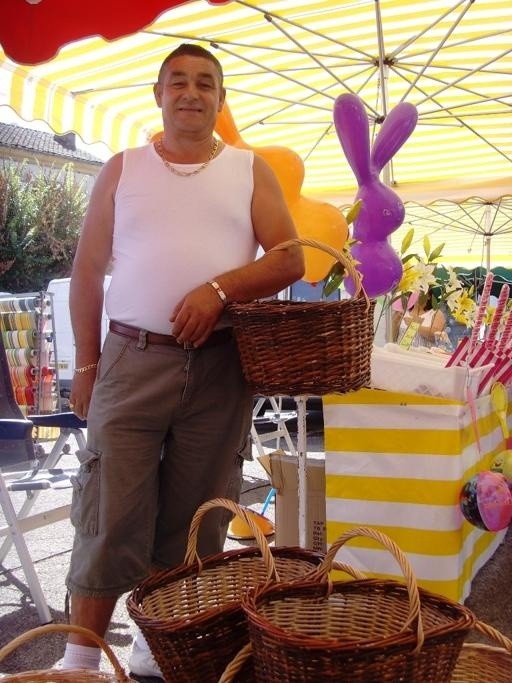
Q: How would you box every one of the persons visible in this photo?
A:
[45,41,302,679]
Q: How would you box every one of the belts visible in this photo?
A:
[109,319,233,350]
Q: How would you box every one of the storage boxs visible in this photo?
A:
[257,448,327,555]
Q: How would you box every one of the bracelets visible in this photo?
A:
[205,280,229,309]
[73,364,97,373]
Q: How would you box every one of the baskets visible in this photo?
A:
[225,238,377,396]
[240,526,477,683]
[0,624,138,683]
[125,498,511,683]
[219,619,512,683]
[126,498,327,683]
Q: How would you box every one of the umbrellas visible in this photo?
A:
[0,1,512,342]
[338,197,512,307]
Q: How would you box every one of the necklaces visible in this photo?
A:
[157,134,219,177]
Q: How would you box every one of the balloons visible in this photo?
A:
[143,90,350,284]
[332,91,419,300]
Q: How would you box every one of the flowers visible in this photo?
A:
[319,198,512,356]
